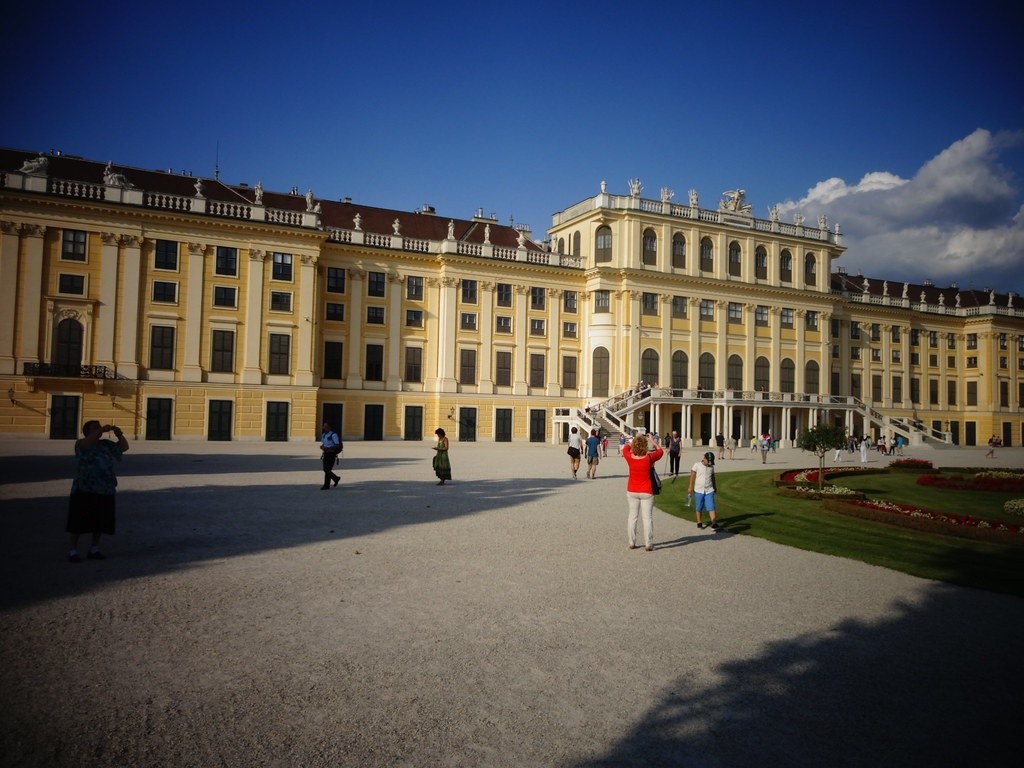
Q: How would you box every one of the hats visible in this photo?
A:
[704,451,716,466]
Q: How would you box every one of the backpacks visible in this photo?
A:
[323,431,344,454]
[988,437,994,445]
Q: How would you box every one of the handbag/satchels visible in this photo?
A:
[650,466,662,495]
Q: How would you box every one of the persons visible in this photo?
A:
[696,383,704,398]
[760,384,765,398]
[601,436,607,458]
[62,420,129,558]
[751,436,757,452]
[585,401,592,413]
[841,435,904,456]
[833,445,842,462]
[584,429,603,480]
[687,452,721,530]
[622,433,664,550]
[725,434,737,461]
[858,435,868,464]
[666,431,683,477]
[766,432,776,454]
[760,432,770,464]
[432,428,452,485]
[638,380,658,399]
[319,423,340,491]
[647,432,656,453]
[664,432,671,449]
[567,427,583,481]
[716,431,725,460]
[984,434,1002,459]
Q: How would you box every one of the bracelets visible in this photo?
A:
[117,431,124,438]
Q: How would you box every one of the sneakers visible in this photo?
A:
[711,523,719,531]
[696,521,703,530]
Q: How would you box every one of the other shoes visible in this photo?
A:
[763,462,767,464]
[721,457,725,459]
[436,481,445,485]
[587,471,595,479]
[321,485,330,490]
[669,473,674,476]
[718,457,721,460]
[89,551,104,560]
[832,459,836,463]
[897,453,900,456]
[865,460,868,462]
[730,457,731,459]
[68,552,81,564]
[733,458,735,460]
[900,454,904,456]
[645,546,653,552]
[861,459,864,462]
[992,457,994,459]
[572,473,577,480]
[334,476,341,487]
[985,454,987,459]
[629,544,635,550]
[882,454,884,456]
